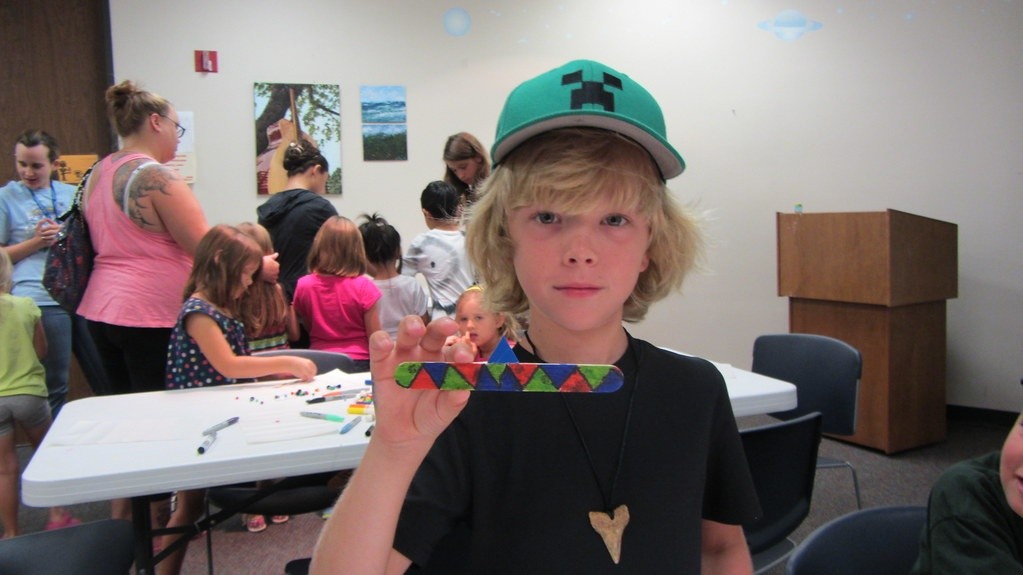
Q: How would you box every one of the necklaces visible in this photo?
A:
[525,324,641,561]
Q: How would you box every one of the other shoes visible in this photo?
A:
[45,513,81,531]
[270,514,289,524]
[242,513,267,532]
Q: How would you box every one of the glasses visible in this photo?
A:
[159,114,187,140]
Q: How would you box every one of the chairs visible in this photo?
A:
[1,332,929,575]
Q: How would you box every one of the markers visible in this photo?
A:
[365,422,376,436]
[306,388,367,404]
[300,409,344,424]
[346,393,374,415]
[198,433,216,454]
[340,416,362,434]
[201,415,240,436]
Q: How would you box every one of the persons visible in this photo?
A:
[0,247,81,539]
[74,81,279,519]
[305,59,756,575]
[910,412,1023,575]
[189,133,515,536]
[157,223,318,575]
[0,126,80,421]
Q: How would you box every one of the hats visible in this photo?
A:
[489,59,687,186]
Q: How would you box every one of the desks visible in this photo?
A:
[21,344,798,575]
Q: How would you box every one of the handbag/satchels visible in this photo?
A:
[42,159,101,314]
